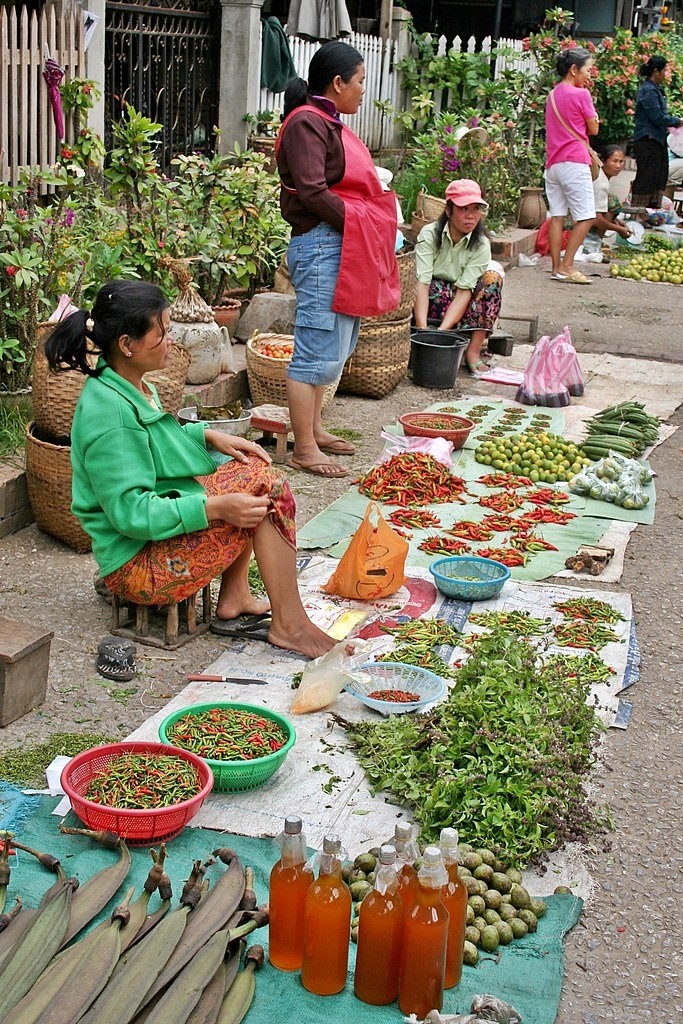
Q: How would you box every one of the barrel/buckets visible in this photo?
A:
[178,405,253,437]
[410,328,469,388]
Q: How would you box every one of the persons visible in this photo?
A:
[563,145,633,240]
[629,55,683,229]
[45,280,354,661]
[274,42,397,475]
[544,49,597,284]
[412,181,505,375]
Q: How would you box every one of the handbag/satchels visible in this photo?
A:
[318,500,408,601]
[513,326,586,407]
[588,149,603,180]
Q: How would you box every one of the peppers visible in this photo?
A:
[350,405,577,581]
[85,709,289,809]
[366,595,627,703]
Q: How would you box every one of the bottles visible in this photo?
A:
[268,817,351,995]
[354,823,468,1020]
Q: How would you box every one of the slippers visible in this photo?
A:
[211,612,276,641]
[287,450,348,478]
[95,636,138,682]
[466,357,490,376]
[555,271,592,284]
[550,274,559,280]
[318,436,356,456]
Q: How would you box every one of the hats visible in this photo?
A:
[445,179,490,209]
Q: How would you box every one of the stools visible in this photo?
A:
[247,403,296,464]
[0,617,55,727]
[110,583,214,651]
[487,315,539,357]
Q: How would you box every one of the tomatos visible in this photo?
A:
[259,343,292,360]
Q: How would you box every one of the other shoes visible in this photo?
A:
[639,220,653,229]
[645,218,661,226]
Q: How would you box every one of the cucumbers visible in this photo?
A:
[578,402,664,462]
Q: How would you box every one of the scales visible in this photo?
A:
[616,207,646,247]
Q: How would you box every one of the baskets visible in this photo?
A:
[429,555,511,600]
[60,741,214,850]
[399,412,476,453]
[157,701,296,795]
[22,183,443,557]
[343,661,446,716]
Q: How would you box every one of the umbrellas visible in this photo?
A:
[42,42,66,148]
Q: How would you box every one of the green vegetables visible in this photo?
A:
[324,630,608,870]
[613,233,675,254]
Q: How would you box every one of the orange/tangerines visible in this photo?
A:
[474,431,652,508]
[609,247,683,284]
